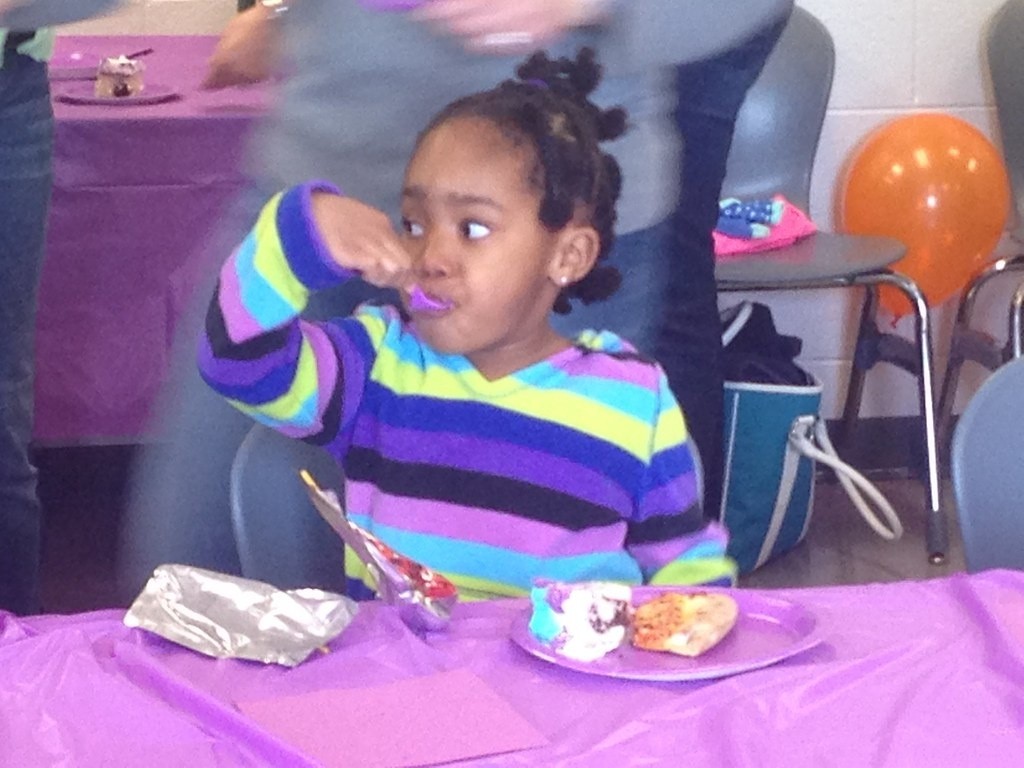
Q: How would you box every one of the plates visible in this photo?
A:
[507,585,837,683]
[59,84,182,104]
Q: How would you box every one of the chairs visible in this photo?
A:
[229,424,351,598]
[716,4,948,563]
[947,360,1019,574]
[925,0,1023,488]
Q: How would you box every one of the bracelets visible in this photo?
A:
[263,0,289,18]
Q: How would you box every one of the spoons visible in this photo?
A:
[402,279,450,315]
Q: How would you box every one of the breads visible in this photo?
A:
[631,589,739,657]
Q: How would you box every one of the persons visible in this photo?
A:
[115,0,796,600]
[0,0,57,611]
[194,46,739,603]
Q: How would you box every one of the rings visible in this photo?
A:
[488,31,529,48]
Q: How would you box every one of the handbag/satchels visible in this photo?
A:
[715,369,902,576]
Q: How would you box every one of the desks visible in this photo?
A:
[30,33,297,450]
[1,571,1023,768]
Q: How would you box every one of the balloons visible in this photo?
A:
[842,115,1013,327]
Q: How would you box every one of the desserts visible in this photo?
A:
[95,53,145,98]
[527,577,631,663]
